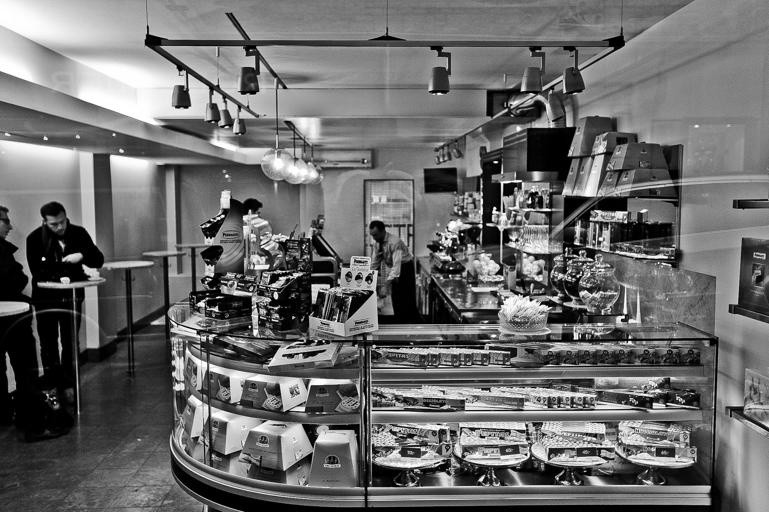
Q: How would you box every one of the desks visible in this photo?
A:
[0,298,31,318]
[175,242,208,295]
[141,250,187,341]
[39,275,107,416]
[101,258,154,377]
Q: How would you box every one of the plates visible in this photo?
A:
[452,441,531,488]
[530,437,616,486]
[373,452,449,488]
[615,438,697,485]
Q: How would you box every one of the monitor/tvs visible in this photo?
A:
[424,167,457,193]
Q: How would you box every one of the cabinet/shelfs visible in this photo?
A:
[169,296,719,512]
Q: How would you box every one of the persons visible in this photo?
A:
[24,200,105,425]
[1,205,72,444]
[244,196,264,217]
[366,219,418,325]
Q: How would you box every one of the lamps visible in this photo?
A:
[316,173,324,183]
[205,103,217,124]
[519,68,549,97]
[426,67,454,95]
[239,67,261,93]
[172,84,192,107]
[303,162,317,182]
[217,108,230,130]
[233,118,245,135]
[287,156,311,184]
[561,47,588,95]
[263,148,292,184]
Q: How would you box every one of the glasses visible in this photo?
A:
[0,218,10,225]
